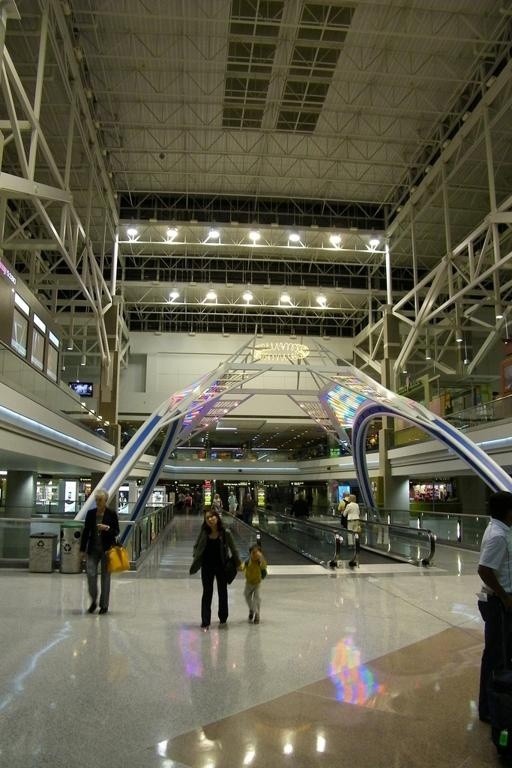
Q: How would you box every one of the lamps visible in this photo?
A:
[60,338,87,383]
[403,305,511,374]
[125,223,380,307]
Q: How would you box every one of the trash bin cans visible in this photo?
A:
[60,521,85,573]
[28,532,58,572]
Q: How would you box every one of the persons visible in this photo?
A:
[475,488,512,767]
[189,623,238,742]
[211,493,224,517]
[338,491,350,529]
[305,491,314,512]
[242,493,253,524]
[194,490,201,513]
[185,494,193,513]
[291,495,309,521]
[228,491,238,516]
[178,490,186,510]
[240,545,267,625]
[189,509,242,628]
[244,626,270,708]
[79,487,121,617]
[341,494,360,550]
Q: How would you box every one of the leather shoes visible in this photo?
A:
[98,607,108,614]
[88,603,97,613]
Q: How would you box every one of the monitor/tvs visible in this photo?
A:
[68,382,93,398]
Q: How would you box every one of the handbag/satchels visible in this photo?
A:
[224,557,236,584]
[105,547,130,573]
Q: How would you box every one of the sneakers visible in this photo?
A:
[249,612,261,624]
